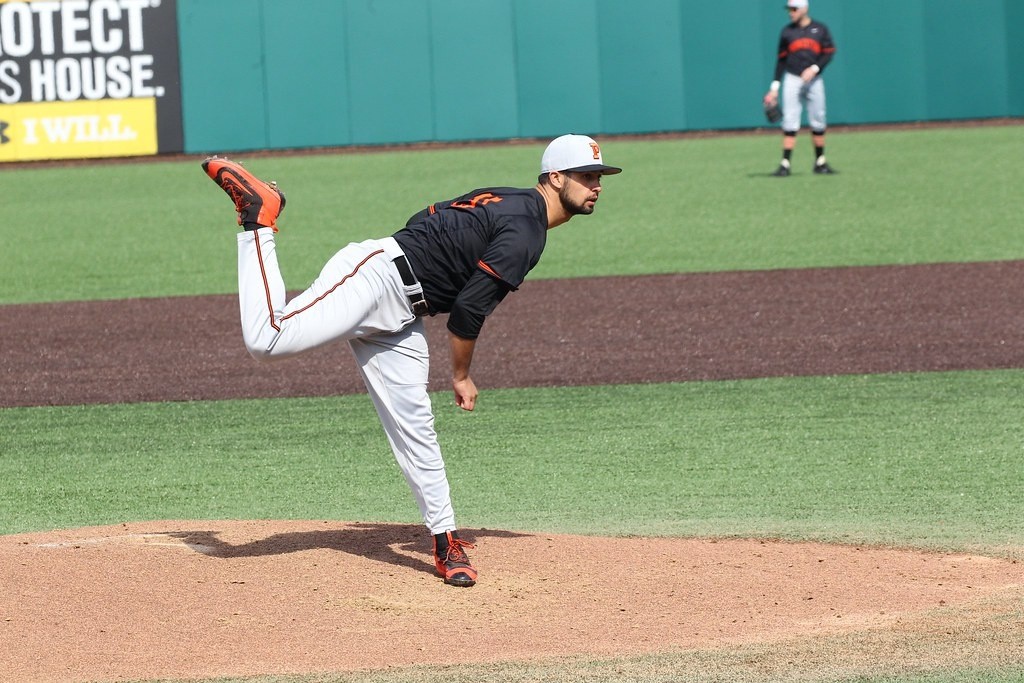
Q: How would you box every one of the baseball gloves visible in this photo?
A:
[764,101,783,124]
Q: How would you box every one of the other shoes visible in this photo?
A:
[813,164,833,175]
[771,165,790,178]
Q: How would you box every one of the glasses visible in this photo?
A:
[788,8,798,12]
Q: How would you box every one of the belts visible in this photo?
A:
[395,255,428,318]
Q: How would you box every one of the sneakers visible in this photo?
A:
[201,154,286,233]
[432,532,477,587]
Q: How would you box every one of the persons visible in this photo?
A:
[765,0,835,176]
[202,133,622,587]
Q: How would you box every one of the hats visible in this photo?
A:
[784,0,808,11]
[541,133,622,175]
